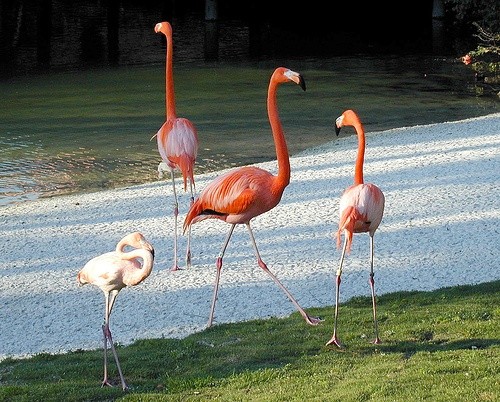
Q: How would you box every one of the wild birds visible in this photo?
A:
[182,66,322,326]
[150,21,201,273]
[324,109,385,349]
[77,232,154,391]
